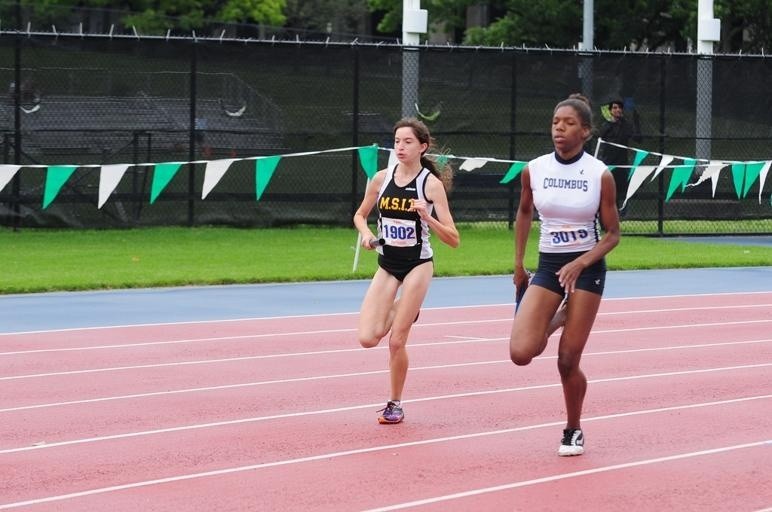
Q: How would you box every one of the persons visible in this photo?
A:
[508,93,621,455]
[599,101,633,217]
[353,117,460,424]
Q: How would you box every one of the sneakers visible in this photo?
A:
[376,401,404,424]
[557,428,585,456]
